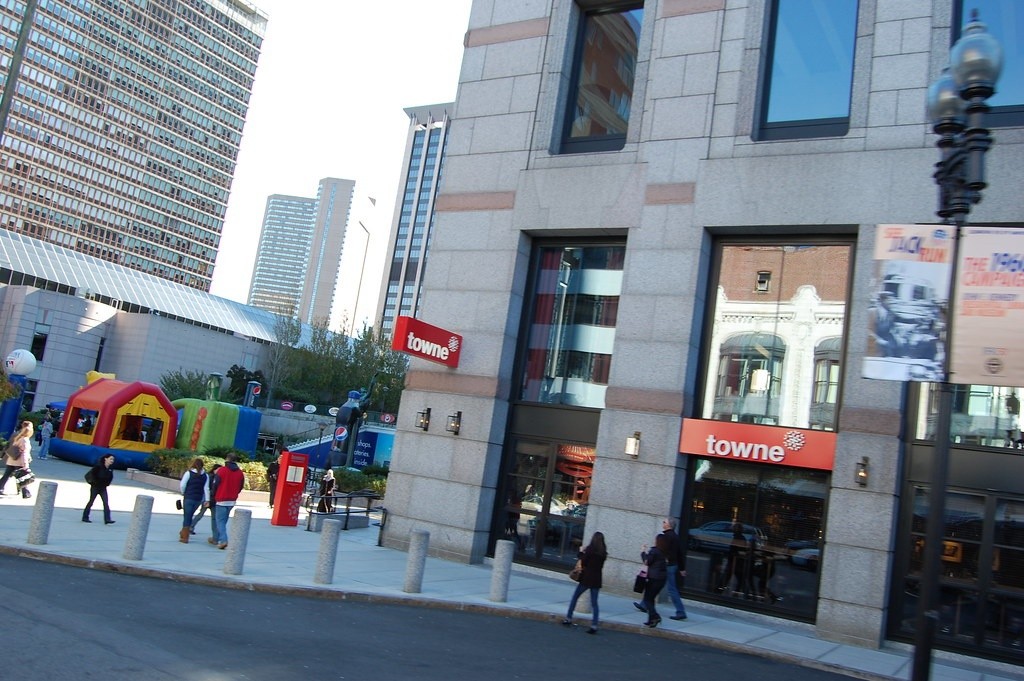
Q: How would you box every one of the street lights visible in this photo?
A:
[308,422,328,490]
[349,220,371,340]
[898,5,1007,679]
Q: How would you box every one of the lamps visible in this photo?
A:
[853,454,870,487]
[416,406,461,435]
[624,430,642,461]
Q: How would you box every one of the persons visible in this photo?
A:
[720,522,785,602]
[188,464,222,538]
[78,415,91,434]
[37,412,64,460]
[0,421,34,499]
[506,495,521,537]
[317,469,336,513]
[180,458,210,543]
[633,518,688,620]
[265,455,282,508]
[866,291,903,358]
[562,532,609,634]
[82,454,116,525]
[265,445,270,452]
[208,452,244,549]
[640,534,667,629]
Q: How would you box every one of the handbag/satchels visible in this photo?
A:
[568,554,583,582]
[5,437,27,460]
[15,468,35,488]
[85,464,101,485]
[633,569,648,593]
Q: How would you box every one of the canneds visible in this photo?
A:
[206,372,223,401]
[243,381,262,409]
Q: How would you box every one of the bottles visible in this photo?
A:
[323,391,360,469]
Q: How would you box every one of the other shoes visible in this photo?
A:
[562,618,572,626]
[208,537,218,545]
[0,489,6,495]
[634,600,646,612]
[670,613,687,620]
[585,627,596,634]
[105,520,115,524]
[38,455,48,461]
[189,527,196,534]
[644,616,662,628]
[219,541,228,549]
[83,518,92,523]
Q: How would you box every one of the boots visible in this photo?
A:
[180,527,189,543]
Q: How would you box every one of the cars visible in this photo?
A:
[785,539,820,569]
[518,492,591,537]
[688,521,768,556]
